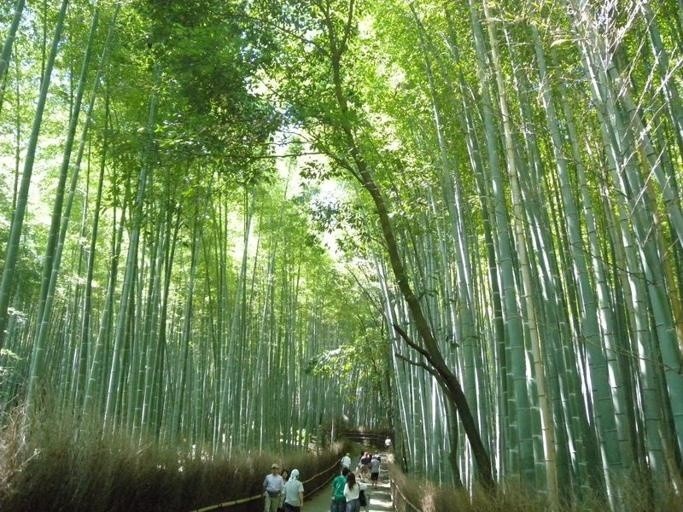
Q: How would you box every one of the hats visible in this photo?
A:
[271,463,279,468]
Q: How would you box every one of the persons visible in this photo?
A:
[281,469,304,512]
[278,470,288,512]
[384,436,391,450]
[263,464,283,512]
[330,449,381,512]
